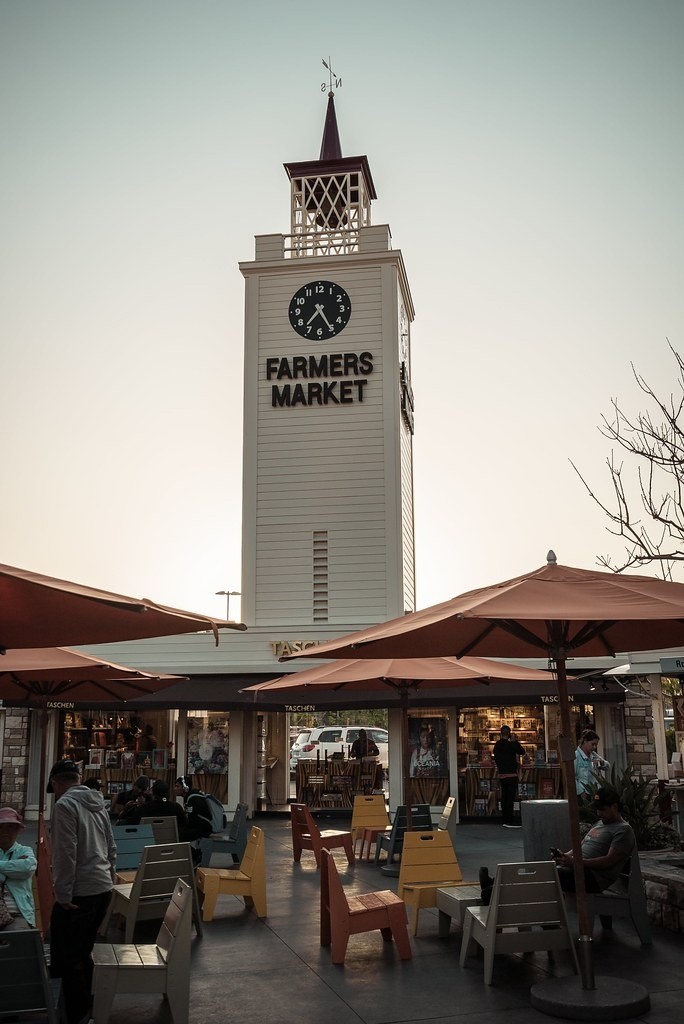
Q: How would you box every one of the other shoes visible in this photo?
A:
[506,818,523,828]
[479,867,493,902]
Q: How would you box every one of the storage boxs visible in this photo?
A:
[85,748,169,769]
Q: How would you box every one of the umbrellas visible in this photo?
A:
[0,648,190,843]
[238,657,577,826]
[0,563,248,654]
[280,550,684,988]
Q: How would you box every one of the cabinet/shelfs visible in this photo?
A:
[67,727,114,752]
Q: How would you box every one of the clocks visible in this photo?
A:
[288,282,351,340]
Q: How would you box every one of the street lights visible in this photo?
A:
[215,590,241,621]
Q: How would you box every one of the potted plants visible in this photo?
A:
[579,768,680,855]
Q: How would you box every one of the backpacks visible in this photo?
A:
[184,793,228,834]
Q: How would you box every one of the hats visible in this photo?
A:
[588,788,620,808]
[45,759,80,793]
[135,775,149,792]
[0,807,25,829]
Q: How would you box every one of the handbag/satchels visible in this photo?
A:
[0,898,15,931]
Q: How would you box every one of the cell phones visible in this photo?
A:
[550,848,562,858]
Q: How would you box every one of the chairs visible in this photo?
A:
[0,794,653,1024]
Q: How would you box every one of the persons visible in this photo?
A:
[45,760,117,1024]
[83,775,212,868]
[140,725,157,751]
[350,729,379,760]
[573,730,610,823]
[494,725,525,828]
[480,788,634,903]
[0,807,37,930]
[410,721,443,777]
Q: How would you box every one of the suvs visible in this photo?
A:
[290,725,389,775]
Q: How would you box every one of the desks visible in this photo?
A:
[465,718,536,745]
[359,826,392,861]
[115,871,137,926]
[435,885,484,938]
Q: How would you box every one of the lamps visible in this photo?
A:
[589,681,596,690]
[602,684,610,691]
